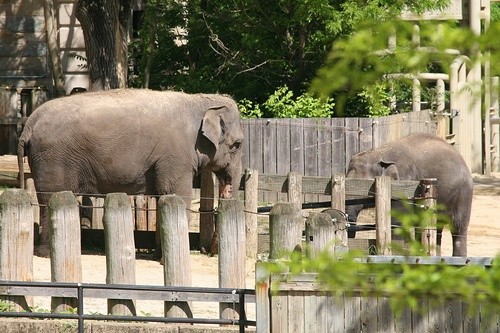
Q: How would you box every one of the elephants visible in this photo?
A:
[17,87,245,261]
[345,131,473,256]
